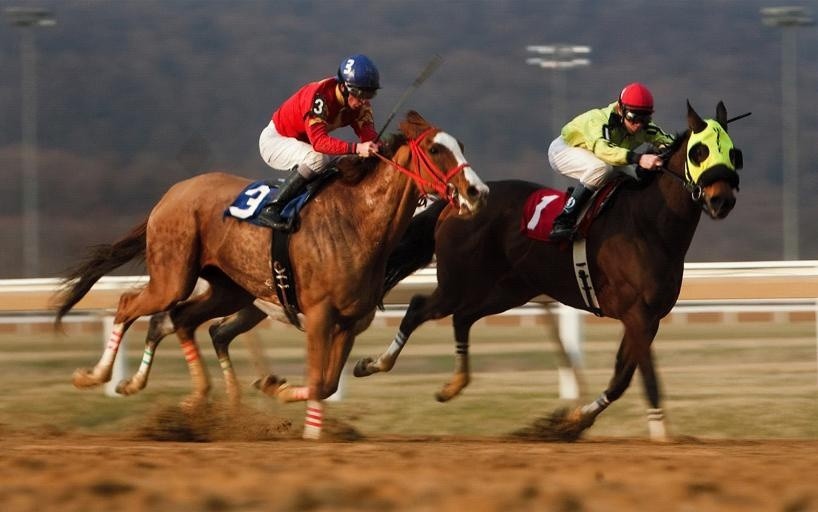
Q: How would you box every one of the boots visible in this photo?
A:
[547,183,593,241]
[257,167,307,229]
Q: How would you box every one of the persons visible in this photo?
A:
[255,55,383,232]
[543,82,678,245]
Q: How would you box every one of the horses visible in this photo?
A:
[352,97,744,446]
[50,110,490,443]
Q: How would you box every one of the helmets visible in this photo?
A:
[619,82,654,114]
[336,53,381,91]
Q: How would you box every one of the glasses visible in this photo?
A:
[353,89,378,99]
[626,110,651,123]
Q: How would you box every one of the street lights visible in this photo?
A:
[759,4,815,262]
[525,40,592,199]
[4,5,57,277]
